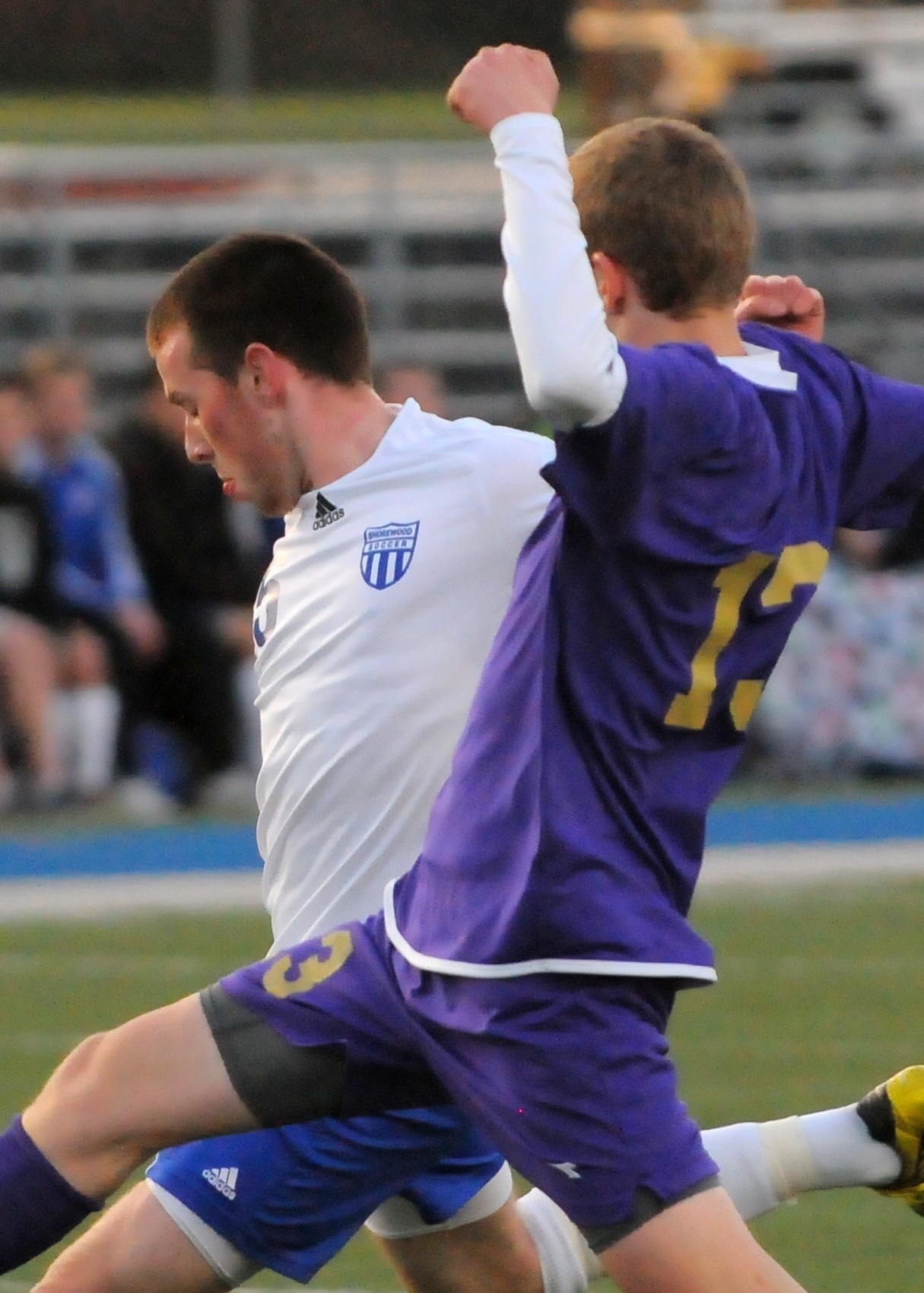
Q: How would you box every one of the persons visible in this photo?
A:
[0,356,281,827]
[0,43,924,1293]
[571,0,726,128]
[30,232,924,1293]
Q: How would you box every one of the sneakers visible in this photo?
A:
[857,1065,924,1218]
